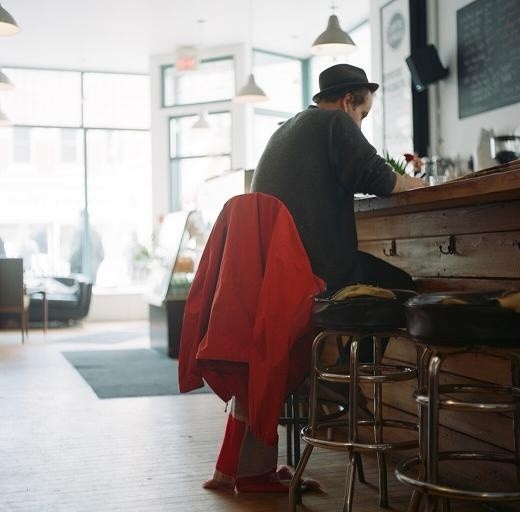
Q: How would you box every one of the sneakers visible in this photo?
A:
[318,376,372,417]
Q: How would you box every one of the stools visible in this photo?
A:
[287,283,423,512]
[404,290,520,511]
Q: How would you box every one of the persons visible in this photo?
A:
[250,64,426,418]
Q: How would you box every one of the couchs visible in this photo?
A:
[22,274,93,329]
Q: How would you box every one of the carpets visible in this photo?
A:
[59,348,214,399]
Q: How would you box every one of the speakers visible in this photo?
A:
[406,43,449,92]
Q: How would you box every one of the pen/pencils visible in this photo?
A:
[421,172,426,178]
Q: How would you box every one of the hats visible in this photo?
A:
[312,64,379,103]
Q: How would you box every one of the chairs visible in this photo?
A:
[0,259,49,345]
[177,193,365,511]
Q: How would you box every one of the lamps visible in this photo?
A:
[309,0,360,59]
[1,70,14,92]
[0,0,22,38]
[229,0,272,105]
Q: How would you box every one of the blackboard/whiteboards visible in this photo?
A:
[456,0,520,120]
